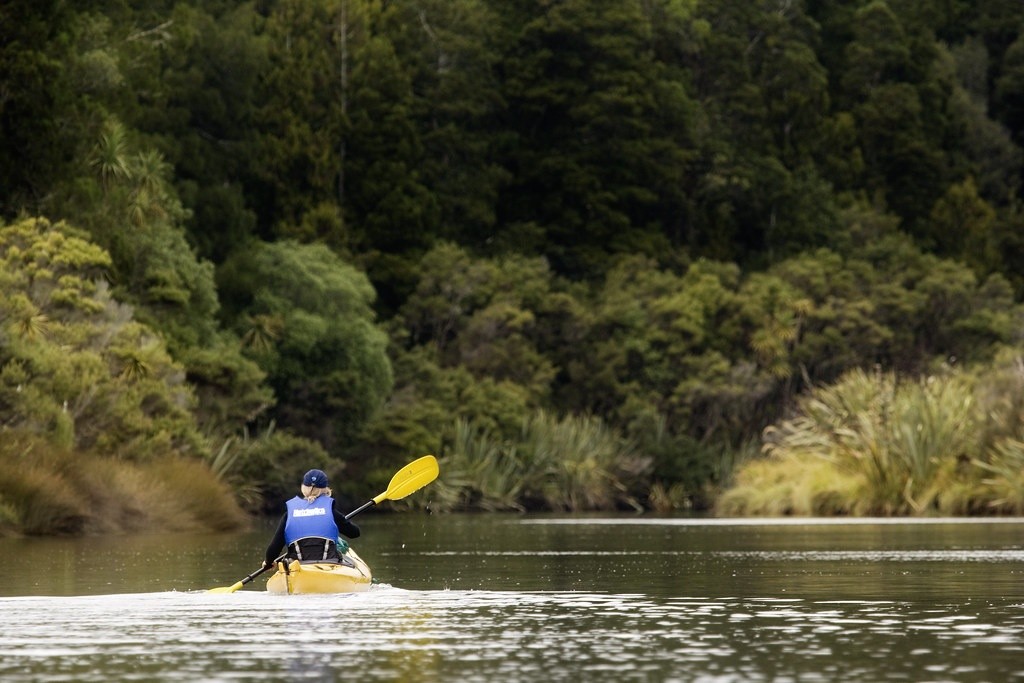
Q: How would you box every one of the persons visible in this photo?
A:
[263,468,361,571]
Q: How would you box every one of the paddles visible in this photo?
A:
[211,455,439,592]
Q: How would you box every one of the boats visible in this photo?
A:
[267,546,371,595]
